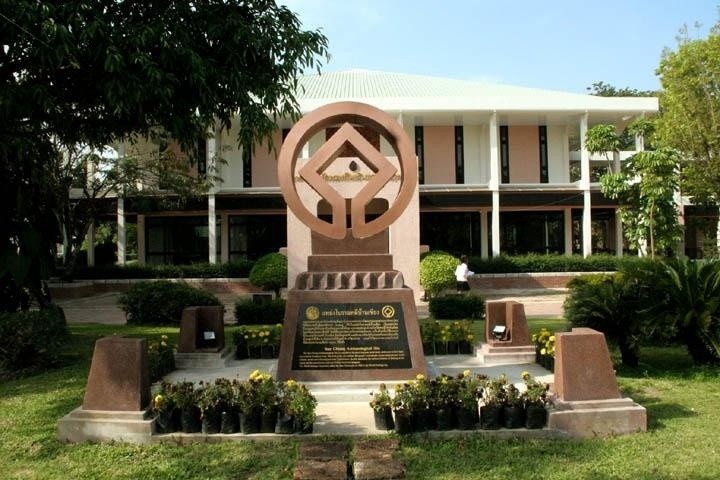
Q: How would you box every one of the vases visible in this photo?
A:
[532,327,557,360]
[232,322,282,347]
[419,319,475,344]
[367,369,555,418]
[153,368,318,428]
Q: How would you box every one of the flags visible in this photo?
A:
[534,349,555,372]
[422,340,472,355]
[237,344,280,360]
[374,401,548,434]
[155,406,314,435]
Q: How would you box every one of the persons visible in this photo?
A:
[454,254,471,293]
